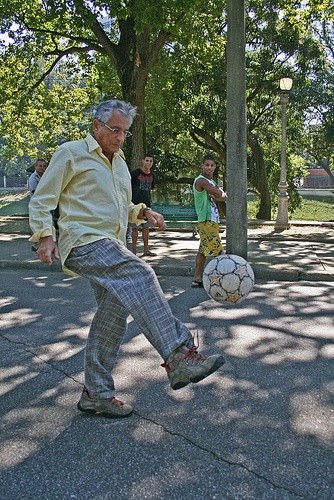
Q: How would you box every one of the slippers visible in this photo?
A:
[144,252,156,256]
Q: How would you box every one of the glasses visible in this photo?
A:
[104,123,133,137]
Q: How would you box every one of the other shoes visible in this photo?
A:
[32,245,39,251]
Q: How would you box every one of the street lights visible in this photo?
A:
[272,74,294,233]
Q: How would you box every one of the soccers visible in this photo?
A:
[202,253,255,305]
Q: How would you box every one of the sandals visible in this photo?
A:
[191,280,204,288]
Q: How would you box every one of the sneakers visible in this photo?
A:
[77,384,133,417]
[159,326,226,390]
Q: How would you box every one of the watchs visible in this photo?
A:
[141,207,151,216]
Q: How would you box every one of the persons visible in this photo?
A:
[28,100,226,419]
[130,153,157,256]
[190,156,226,289]
[28,159,47,251]
